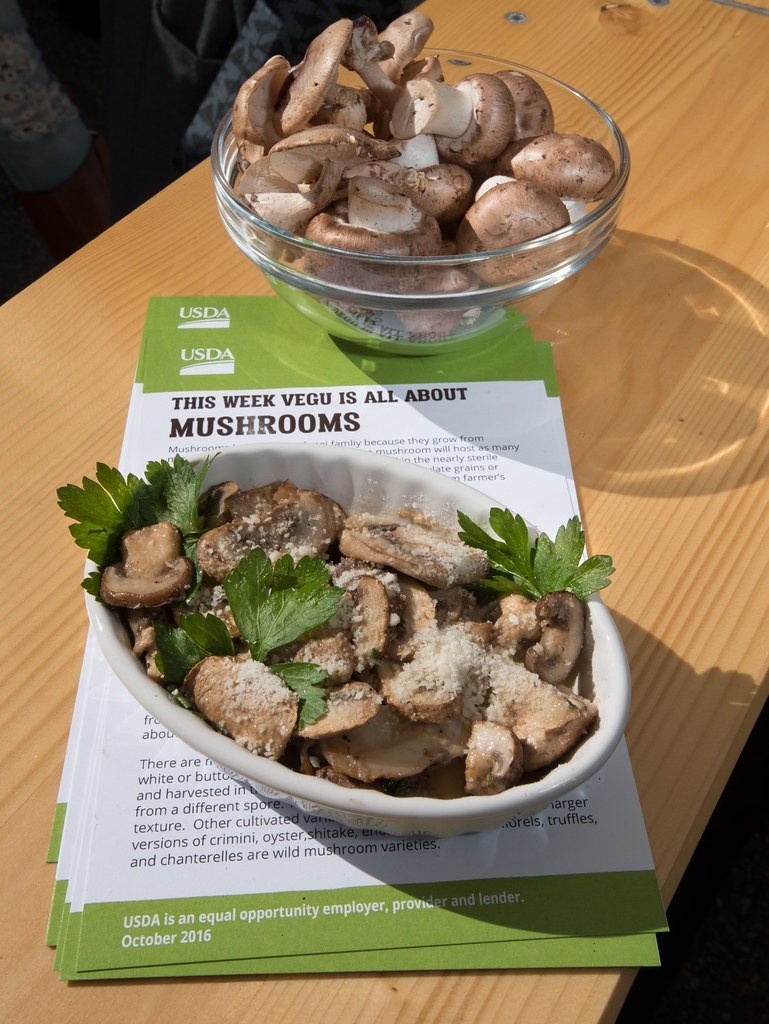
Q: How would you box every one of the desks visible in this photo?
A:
[0,0,769,1024]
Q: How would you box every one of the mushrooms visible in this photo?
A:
[232,14,617,333]
[100,481,586,794]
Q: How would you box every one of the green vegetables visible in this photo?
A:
[55,451,617,730]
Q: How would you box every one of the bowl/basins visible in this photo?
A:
[84,441,633,834]
[210,46,632,356]
